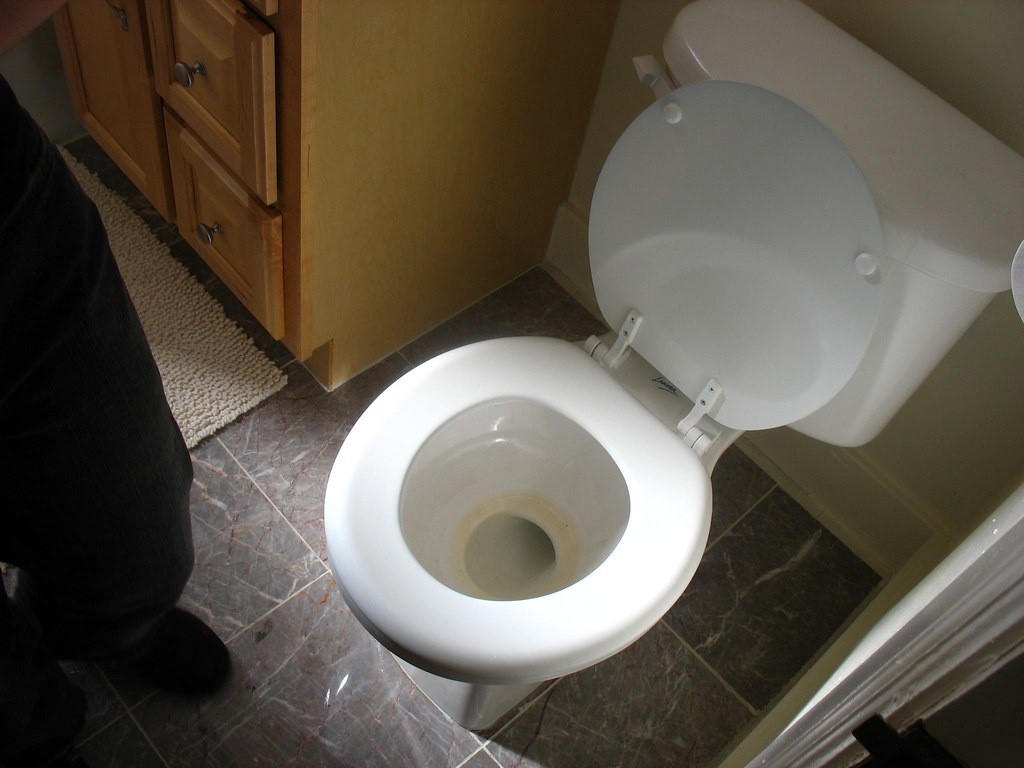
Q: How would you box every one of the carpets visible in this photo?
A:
[53,144,287,450]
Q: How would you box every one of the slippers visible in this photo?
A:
[87,606,230,698]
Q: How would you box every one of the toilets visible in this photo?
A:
[322,3,1023,734]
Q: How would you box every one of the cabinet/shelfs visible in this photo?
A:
[49,0,617,392]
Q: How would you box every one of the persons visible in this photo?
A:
[2,0,233,768]
